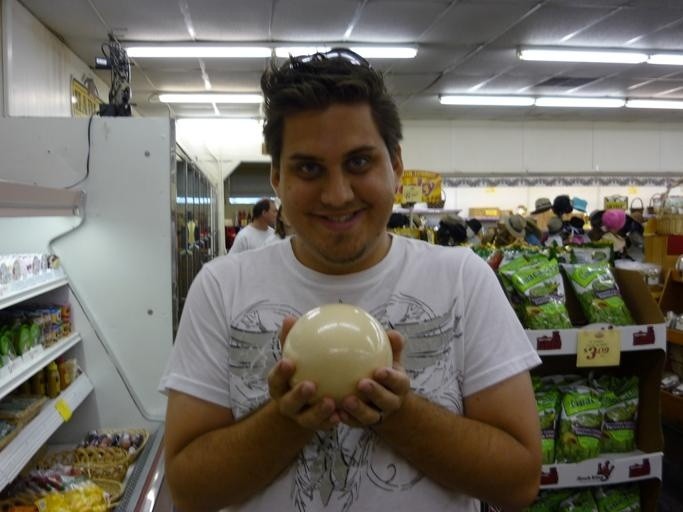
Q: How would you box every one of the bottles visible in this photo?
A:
[18,356,68,399]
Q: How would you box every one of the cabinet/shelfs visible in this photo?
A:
[0,217,171,512]
[517,267,683,490]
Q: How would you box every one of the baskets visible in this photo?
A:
[657,204,682,236]
[647,194,662,214]
[629,198,644,212]
[425,188,446,208]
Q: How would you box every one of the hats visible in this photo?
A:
[546,216,564,236]
[570,197,587,213]
[588,209,605,226]
[526,216,537,228]
[550,195,573,215]
[531,197,551,215]
[601,208,626,233]
[563,216,584,235]
[505,214,527,239]
[466,219,482,235]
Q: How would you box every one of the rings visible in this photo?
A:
[371,412,383,427]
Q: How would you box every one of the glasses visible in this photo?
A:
[278,47,372,71]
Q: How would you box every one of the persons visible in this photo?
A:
[170,208,213,253]
[275,205,294,242]
[463,217,482,240]
[159,46,542,512]
[227,199,279,254]
